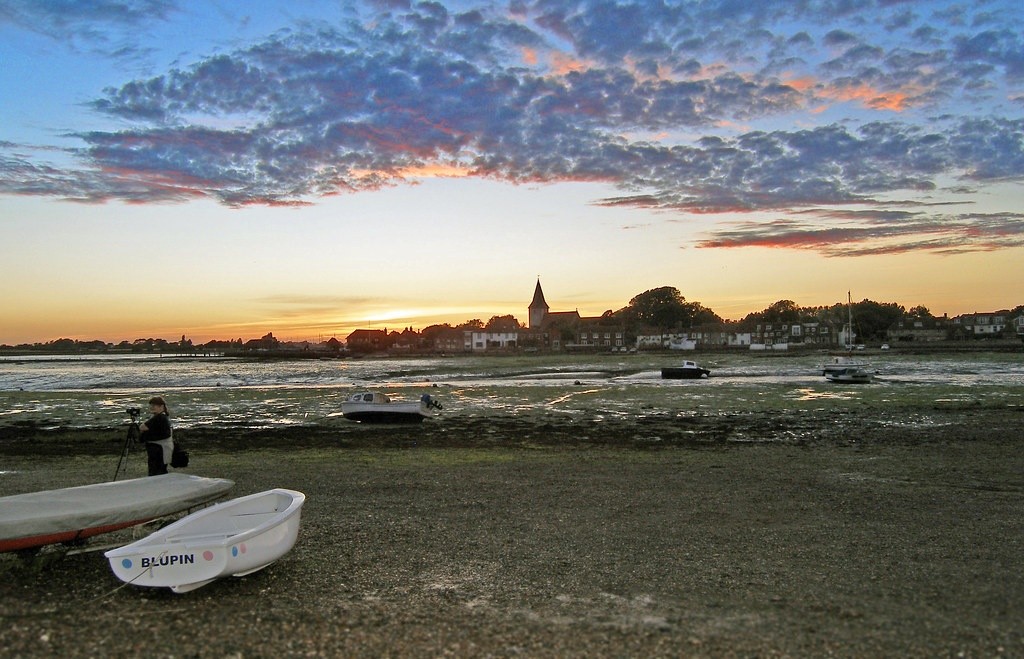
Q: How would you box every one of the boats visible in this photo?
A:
[341,392,444,424]
[104,488,305,593]
[0,472,237,558]
[661,359,710,379]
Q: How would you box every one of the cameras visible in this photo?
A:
[126,407,140,416]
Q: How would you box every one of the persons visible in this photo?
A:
[140,396,175,475]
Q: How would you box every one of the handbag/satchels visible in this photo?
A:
[170,441,189,468]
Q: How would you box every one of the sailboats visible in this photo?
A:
[821,289,877,384]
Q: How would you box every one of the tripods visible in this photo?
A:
[113,416,144,482]
